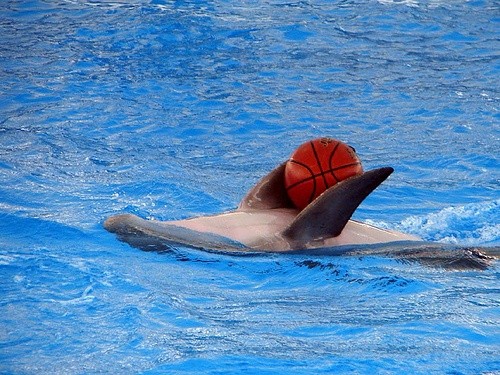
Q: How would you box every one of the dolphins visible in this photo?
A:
[102,143,500,271]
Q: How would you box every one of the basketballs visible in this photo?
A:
[284,137,363,211]
